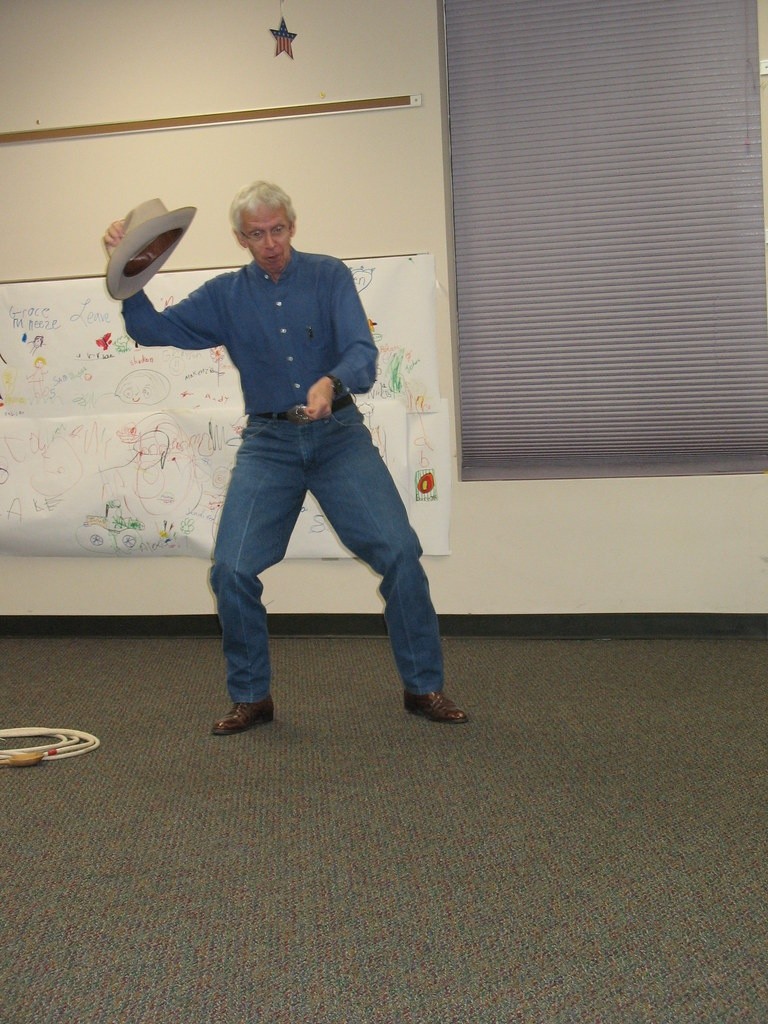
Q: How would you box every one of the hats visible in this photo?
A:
[106,198,197,300]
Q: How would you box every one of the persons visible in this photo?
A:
[104,181,469,734]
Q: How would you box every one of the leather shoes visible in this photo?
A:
[403,687,467,723]
[214,694,275,734]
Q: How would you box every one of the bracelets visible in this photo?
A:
[326,374,342,395]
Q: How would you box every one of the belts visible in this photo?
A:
[255,394,353,420]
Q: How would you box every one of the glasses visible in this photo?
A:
[240,221,292,241]
